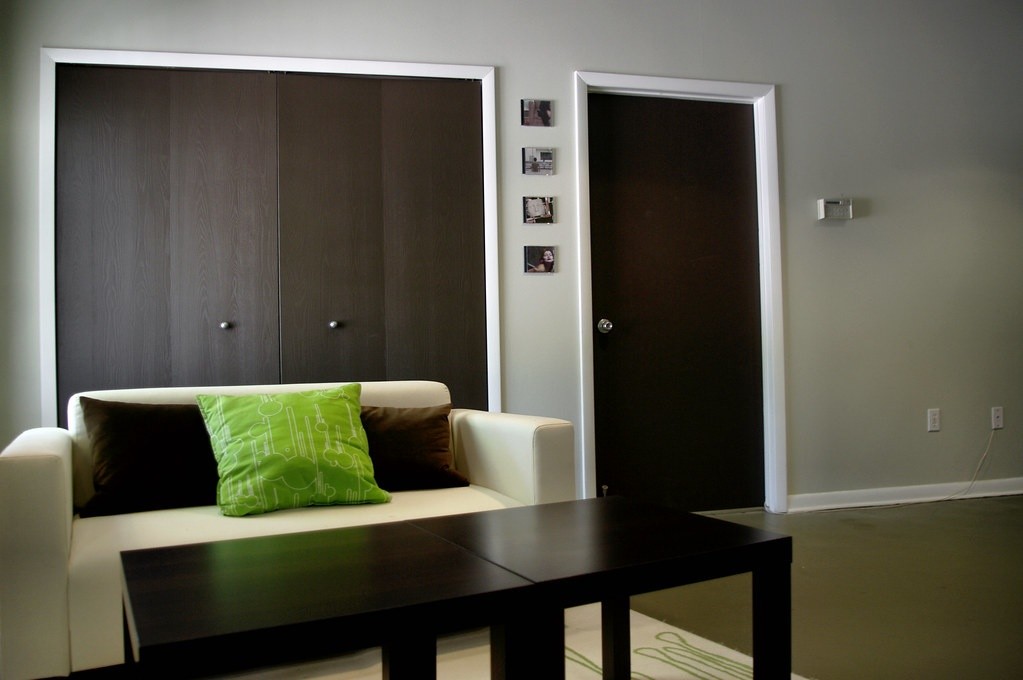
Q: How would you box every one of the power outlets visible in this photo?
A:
[992,407,1003,430]
[927,408,940,431]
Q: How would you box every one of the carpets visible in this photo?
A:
[206,598,813,680]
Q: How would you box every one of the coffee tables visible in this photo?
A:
[117,495,795,680]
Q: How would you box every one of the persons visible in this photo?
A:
[529,249,554,273]
[530,158,540,172]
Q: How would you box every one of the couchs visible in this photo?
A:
[0,379,577,680]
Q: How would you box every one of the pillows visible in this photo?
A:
[359,401,471,494]
[76,391,215,518]
[193,382,393,516]
